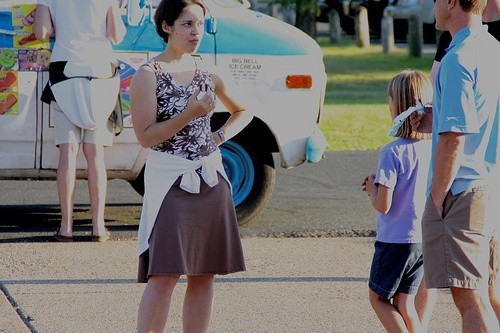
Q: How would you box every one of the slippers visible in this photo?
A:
[90,227,110,243]
[53,226,73,242]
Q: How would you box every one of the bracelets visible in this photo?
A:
[217,129,225,143]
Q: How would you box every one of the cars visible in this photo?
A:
[0,1,327,228]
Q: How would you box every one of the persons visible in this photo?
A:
[130,0,254,333]
[411,0,500,333]
[33,0,126,242]
[361,71,431,333]
